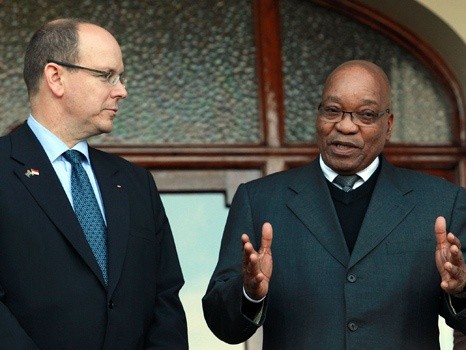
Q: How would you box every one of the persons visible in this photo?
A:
[202,60,466,350]
[1,19,189,350]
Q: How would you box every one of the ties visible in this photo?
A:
[334,174,360,193]
[61,150,111,287]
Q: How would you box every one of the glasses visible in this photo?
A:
[317,102,391,126]
[47,58,128,86]
